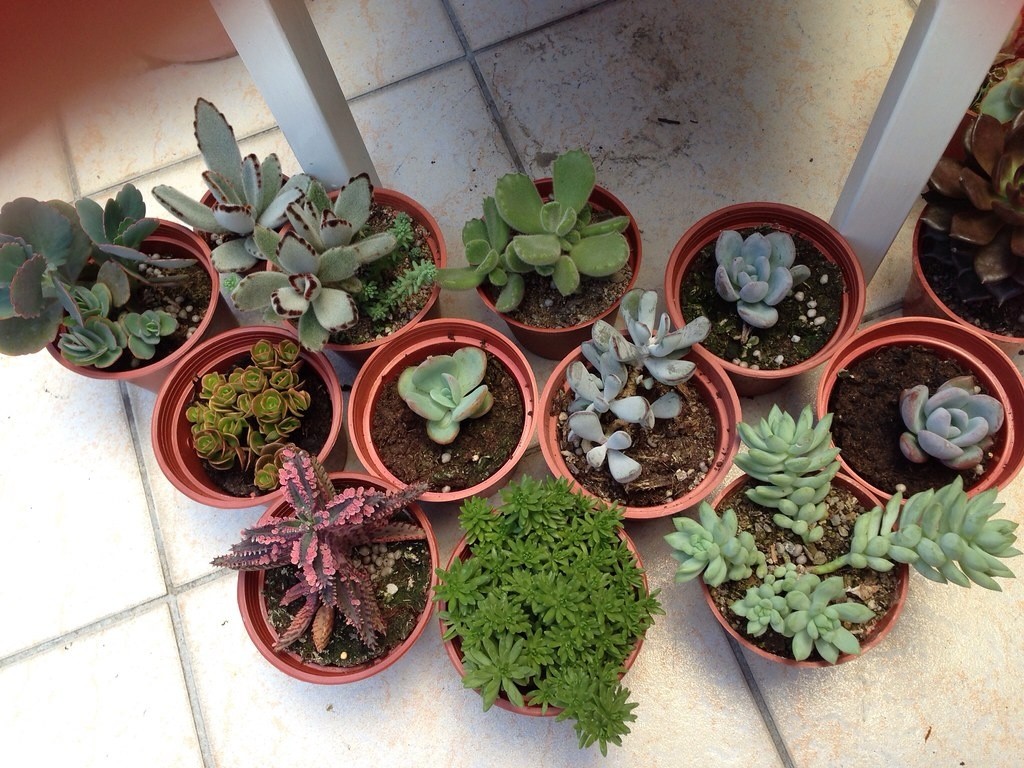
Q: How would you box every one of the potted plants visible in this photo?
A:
[0,183,227,396]
[658,201,868,395]
[151,96,301,273]
[348,318,539,503]
[230,171,448,351]
[436,476,665,759]
[815,313,1024,506]
[945,49,1023,152]
[210,444,441,689]
[535,287,745,517]
[436,150,644,361]
[664,403,1024,669]
[151,326,344,509]
[894,111,1024,363]
